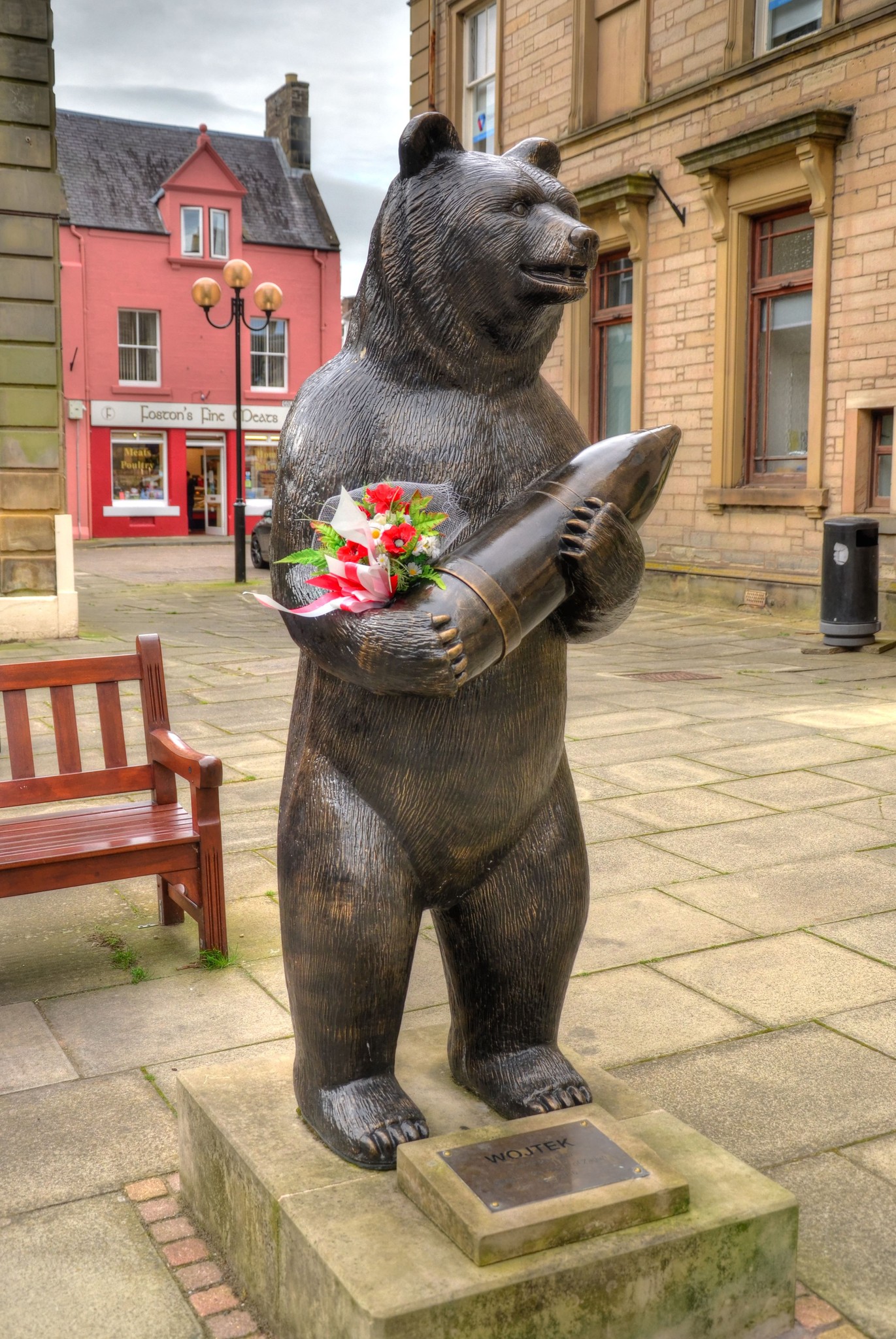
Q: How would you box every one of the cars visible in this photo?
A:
[250,510,274,569]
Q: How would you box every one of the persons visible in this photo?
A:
[258,470,277,497]
[186,470,195,534]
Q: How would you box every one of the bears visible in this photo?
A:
[262,107,684,1179]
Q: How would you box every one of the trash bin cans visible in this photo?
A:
[822,517,880,648]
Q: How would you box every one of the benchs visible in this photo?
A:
[0,632,229,964]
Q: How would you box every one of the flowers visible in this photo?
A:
[273,484,446,591]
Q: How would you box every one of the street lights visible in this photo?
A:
[192,259,285,586]
